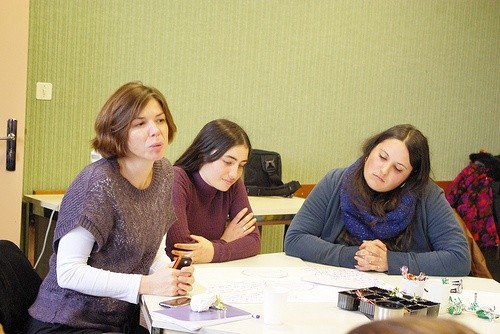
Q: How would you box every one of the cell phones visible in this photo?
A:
[159,296,191,308]
[173,256,192,269]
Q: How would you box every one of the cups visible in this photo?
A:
[373,301,404,320]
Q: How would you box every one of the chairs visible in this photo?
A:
[0,239,43,334]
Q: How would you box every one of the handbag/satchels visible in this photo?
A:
[244,149,301,198]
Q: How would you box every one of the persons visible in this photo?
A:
[284,124,471,276]
[28,81,195,334]
[165,118,261,264]
[369,261,372,263]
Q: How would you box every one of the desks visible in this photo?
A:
[141,253,500,334]
[24,194,304,280]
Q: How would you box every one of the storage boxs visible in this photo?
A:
[337,286,440,319]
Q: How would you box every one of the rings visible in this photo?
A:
[243,226,246,231]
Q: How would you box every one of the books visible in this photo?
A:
[152,300,252,330]
